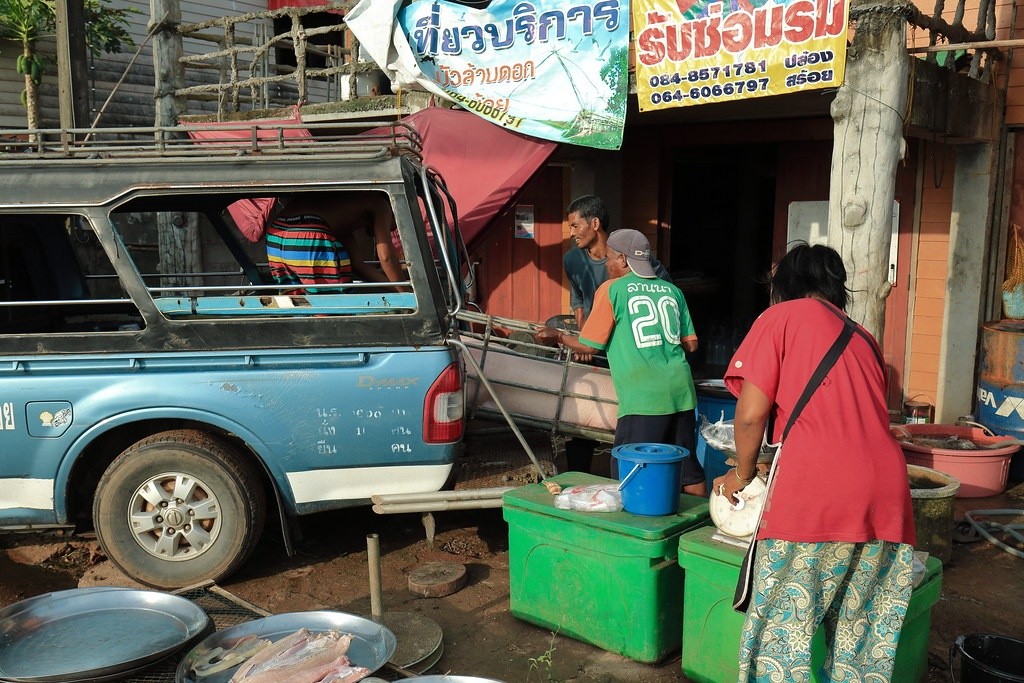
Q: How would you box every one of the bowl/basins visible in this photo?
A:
[891,425,1021,498]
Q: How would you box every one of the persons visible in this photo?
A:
[267,191,410,294]
[713,243,918,683]
[533,229,707,499]
[562,194,672,358]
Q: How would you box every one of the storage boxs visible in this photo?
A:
[501,472,713,664]
[676,526,943,683]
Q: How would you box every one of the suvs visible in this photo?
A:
[1,126,470,587]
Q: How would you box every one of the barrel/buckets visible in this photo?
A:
[949,633,1024,683]
[976,320,1024,479]
[902,402,931,426]
[694,397,739,499]
[611,443,690,516]
[906,464,960,565]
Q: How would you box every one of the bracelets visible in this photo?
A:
[558,332,563,343]
[735,465,757,485]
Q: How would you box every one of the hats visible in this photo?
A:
[607,228,657,278]
